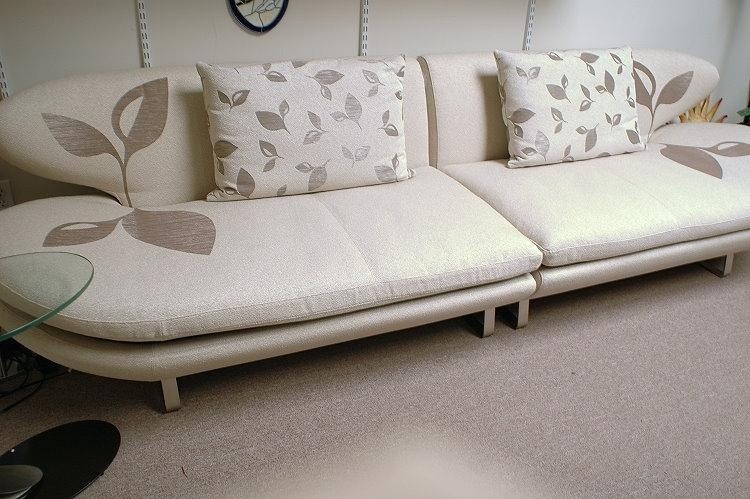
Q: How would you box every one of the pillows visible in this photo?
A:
[196,53,415,205]
[493,46,646,170]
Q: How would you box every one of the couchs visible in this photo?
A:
[0,51,749,411]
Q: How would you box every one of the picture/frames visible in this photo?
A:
[229,0,290,33]
